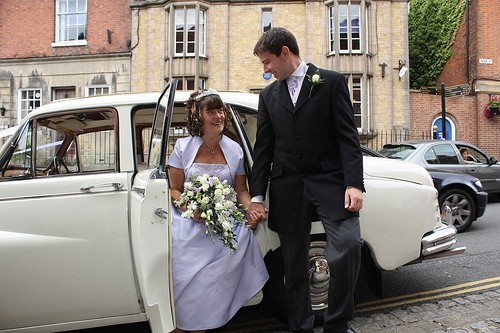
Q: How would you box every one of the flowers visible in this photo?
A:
[304,67,327,98]
[484,94,500,120]
[172,173,253,253]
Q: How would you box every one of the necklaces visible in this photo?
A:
[204,141,218,155]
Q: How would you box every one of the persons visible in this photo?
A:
[459,148,477,164]
[166,89,269,333]
[246,27,367,333]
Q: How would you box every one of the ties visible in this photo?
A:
[286,77,297,108]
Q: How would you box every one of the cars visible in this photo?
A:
[0,79,466,333]
[357,143,489,232]
[378,139,500,194]
